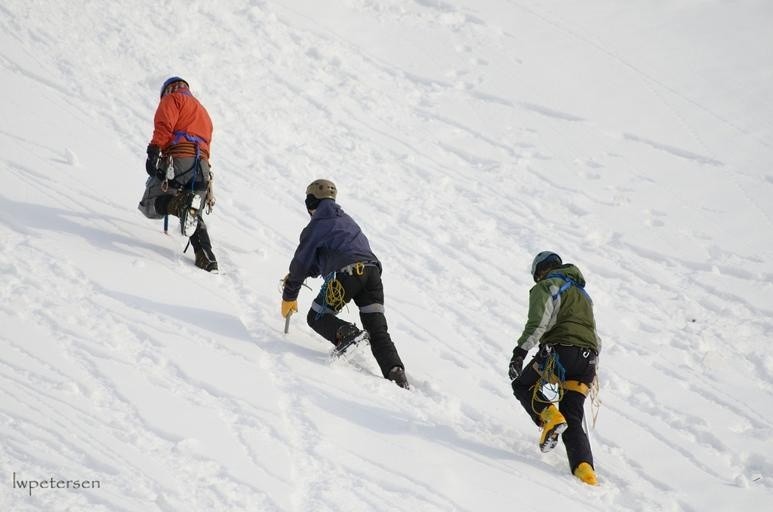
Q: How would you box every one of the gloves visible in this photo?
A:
[281,299,298,319]
[507,345,528,380]
[145,145,160,176]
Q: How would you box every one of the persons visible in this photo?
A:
[508,250,602,485]
[137,76,219,276]
[280,178,410,390]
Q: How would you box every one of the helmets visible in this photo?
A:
[160,77,190,99]
[305,179,337,210]
[530,250,563,283]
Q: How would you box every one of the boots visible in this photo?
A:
[168,192,201,237]
[190,229,219,273]
[538,404,569,453]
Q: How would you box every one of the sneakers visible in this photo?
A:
[387,366,410,390]
[332,324,371,362]
[574,461,597,486]
